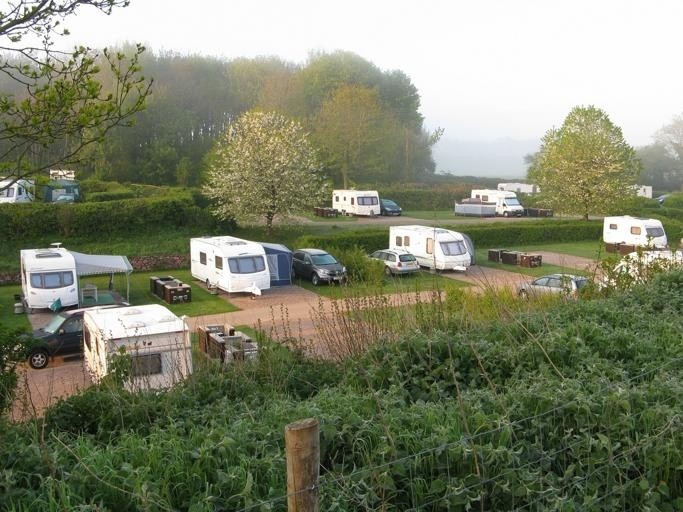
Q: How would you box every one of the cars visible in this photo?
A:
[655,194,667,203]
[52,192,81,205]
[379,199,401,216]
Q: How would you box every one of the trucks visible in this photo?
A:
[469,188,524,218]
[598,250,681,292]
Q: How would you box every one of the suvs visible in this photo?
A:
[9,304,128,368]
[291,248,348,286]
[517,273,590,302]
[363,249,420,275]
